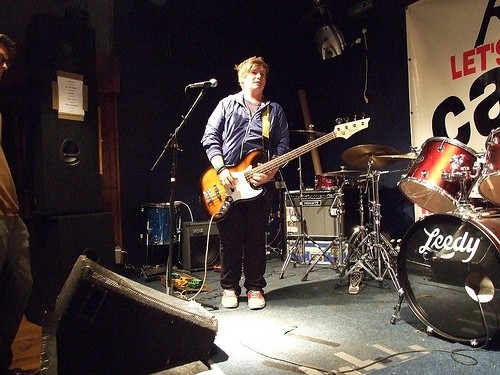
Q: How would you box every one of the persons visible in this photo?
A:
[200,55,289,309]
[0,33,44,328]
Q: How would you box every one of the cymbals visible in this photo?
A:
[375,152,418,160]
[325,170,361,173]
[289,129,328,136]
[340,145,403,172]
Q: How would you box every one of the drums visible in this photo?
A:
[398,137,483,213]
[138,200,182,246]
[397,208,500,344]
[314,174,366,190]
[478,126,500,207]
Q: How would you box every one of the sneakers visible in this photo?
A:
[247,290,265,309]
[221,289,238,307]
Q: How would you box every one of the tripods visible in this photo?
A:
[279,150,401,293]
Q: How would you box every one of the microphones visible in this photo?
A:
[188,78,218,88]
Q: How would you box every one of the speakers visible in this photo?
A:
[23,108,103,216]
[37,213,116,280]
[53,253,217,375]
[284,196,346,241]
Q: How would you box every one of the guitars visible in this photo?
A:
[199,113,371,223]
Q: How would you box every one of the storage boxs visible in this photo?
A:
[284,190,349,266]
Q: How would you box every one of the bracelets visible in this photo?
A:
[216,165,226,174]
